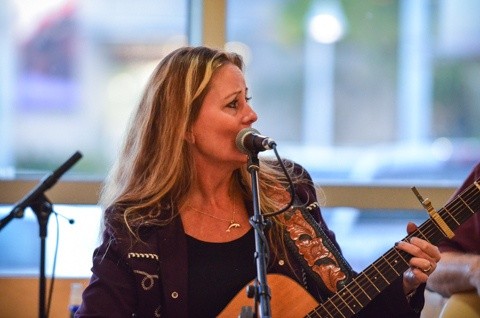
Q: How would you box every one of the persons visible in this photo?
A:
[76,46,440,318]
[425,161,480,318]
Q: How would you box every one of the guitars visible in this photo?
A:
[218,164,480,318]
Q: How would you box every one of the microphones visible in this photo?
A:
[236,127,276,154]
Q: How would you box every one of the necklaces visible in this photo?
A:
[180,190,240,232]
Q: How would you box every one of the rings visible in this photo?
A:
[422,262,433,272]
